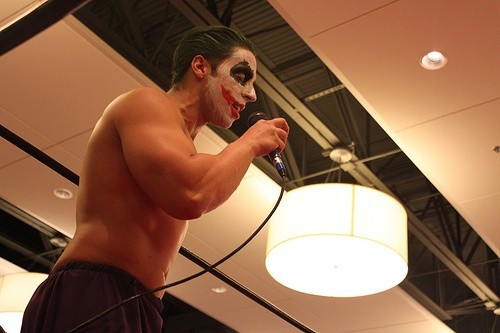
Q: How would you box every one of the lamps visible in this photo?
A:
[264,143,412,299]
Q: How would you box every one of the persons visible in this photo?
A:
[23,24,289,333]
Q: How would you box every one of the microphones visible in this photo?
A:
[247,111,286,177]
